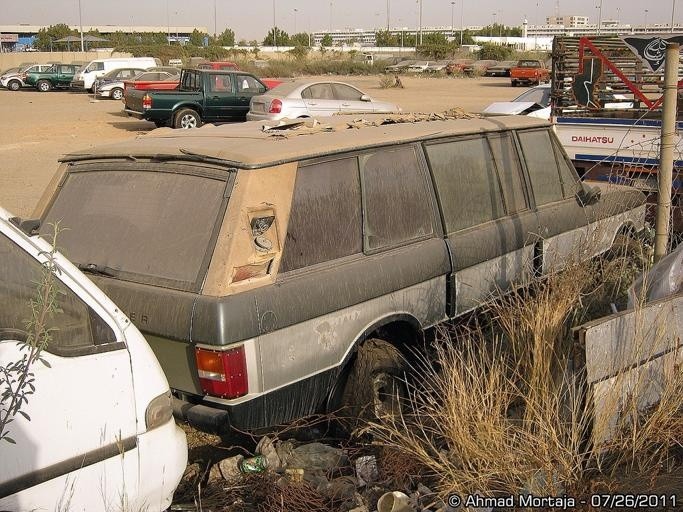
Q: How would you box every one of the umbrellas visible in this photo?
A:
[52,35,110,52]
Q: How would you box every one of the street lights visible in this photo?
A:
[329,1,334,33]
[451,1,456,36]
[645,9,648,27]
[294,8,298,32]
